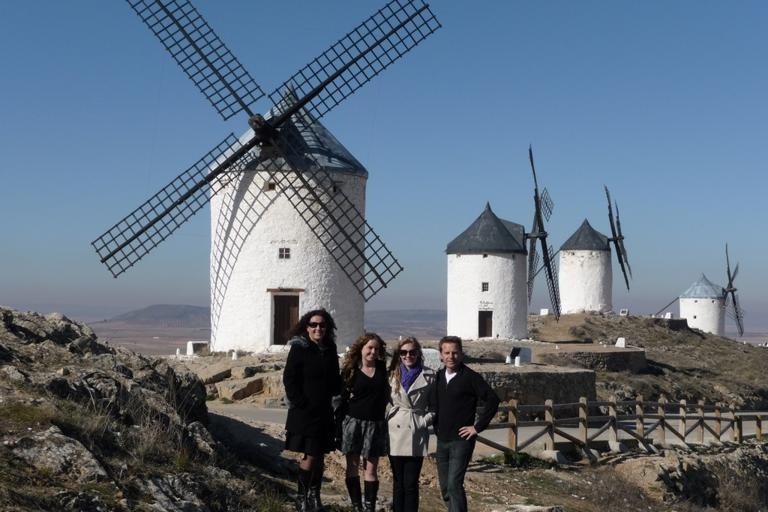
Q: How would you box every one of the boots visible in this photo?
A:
[309,470,324,512]
[364,480,380,512]
[295,468,312,512]
[345,476,363,512]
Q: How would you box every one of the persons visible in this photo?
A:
[283,310,344,512]
[384,336,438,512]
[340,333,390,512]
[436,336,501,512]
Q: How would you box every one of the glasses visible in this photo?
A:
[399,350,418,355]
[307,322,327,328]
